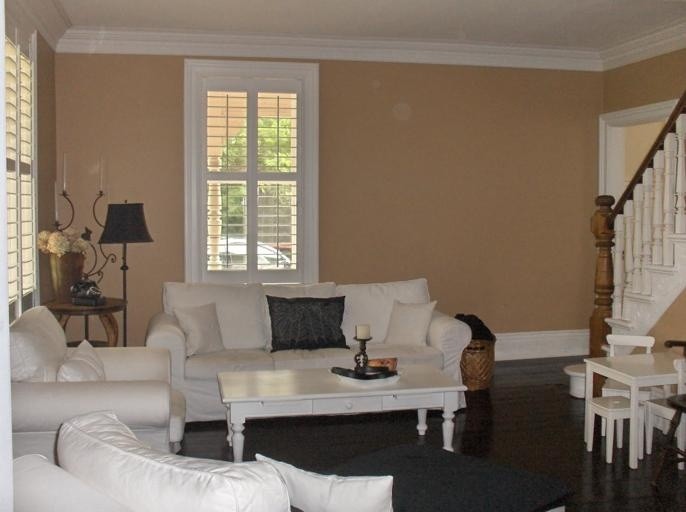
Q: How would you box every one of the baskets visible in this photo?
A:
[369,358,398,373]
[458,341,495,397]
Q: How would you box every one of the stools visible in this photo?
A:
[584,331,685,492]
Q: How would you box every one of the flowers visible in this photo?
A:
[35,228,90,258]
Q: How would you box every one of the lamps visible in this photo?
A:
[97,205,154,347]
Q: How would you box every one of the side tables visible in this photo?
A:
[42,294,127,348]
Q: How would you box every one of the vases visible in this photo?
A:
[49,248,84,304]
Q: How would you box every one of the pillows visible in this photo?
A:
[54,337,105,382]
[264,292,348,353]
[170,302,228,358]
[252,450,394,511]
[383,296,440,349]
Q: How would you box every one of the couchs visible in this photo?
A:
[11,406,575,511]
[143,273,473,425]
[8,297,187,457]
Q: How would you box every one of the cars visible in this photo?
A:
[207,234,292,269]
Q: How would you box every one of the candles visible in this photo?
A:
[100,152,103,192]
[63,152,66,192]
[355,323,371,339]
